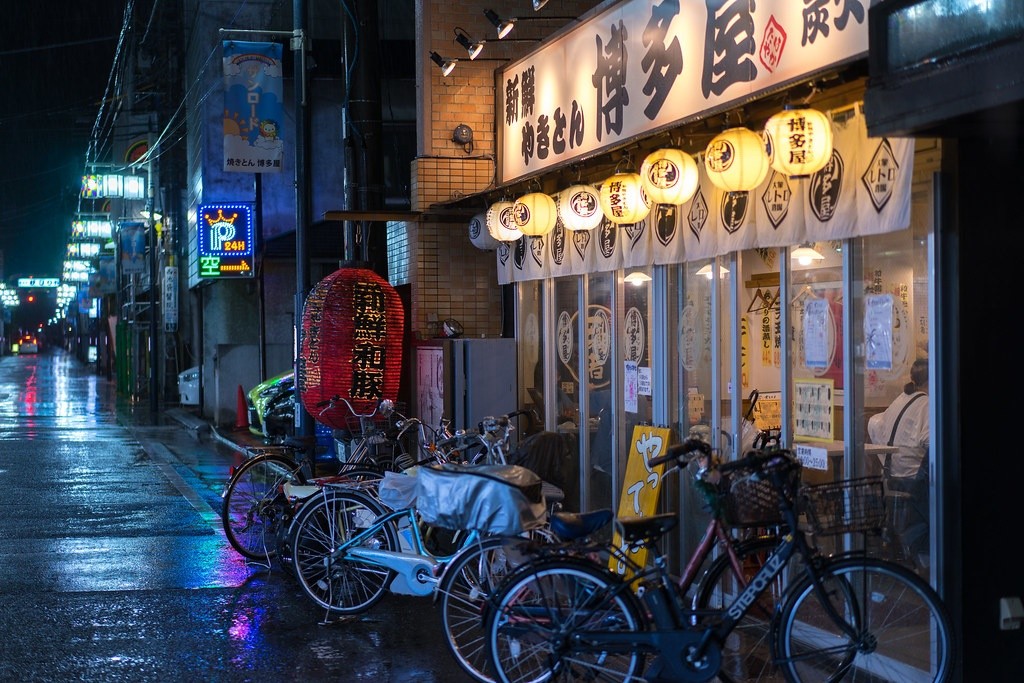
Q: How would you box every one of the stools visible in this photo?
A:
[866,488,911,538]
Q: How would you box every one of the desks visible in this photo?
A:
[795,439,900,555]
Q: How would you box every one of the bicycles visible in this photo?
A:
[220,395,581,643]
[479,450,955,683]
[433,429,861,683]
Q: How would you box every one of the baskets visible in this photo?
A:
[719,454,803,527]
[805,474,888,535]
[348,400,406,438]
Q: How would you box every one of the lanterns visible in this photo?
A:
[468,101,836,251]
[297,258,405,433]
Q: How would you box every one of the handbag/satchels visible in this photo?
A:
[866,475,920,534]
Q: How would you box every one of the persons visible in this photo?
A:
[868,356,931,557]
[587,381,653,486]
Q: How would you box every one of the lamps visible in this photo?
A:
[441,318,465,338]
[482,8,579,40]
[429,50,511,77]
[695,258,731,279]
[454,26,542,61]
[533,0,548,12]
[790,241,825,264]
[624,270,652,286]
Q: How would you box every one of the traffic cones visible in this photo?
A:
[233,385,249,432]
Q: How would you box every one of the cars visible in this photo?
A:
[176,365,204,407]
[246,368,297,439]
[17,336,38,353]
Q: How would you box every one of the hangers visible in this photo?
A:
[746,278,822,314]
